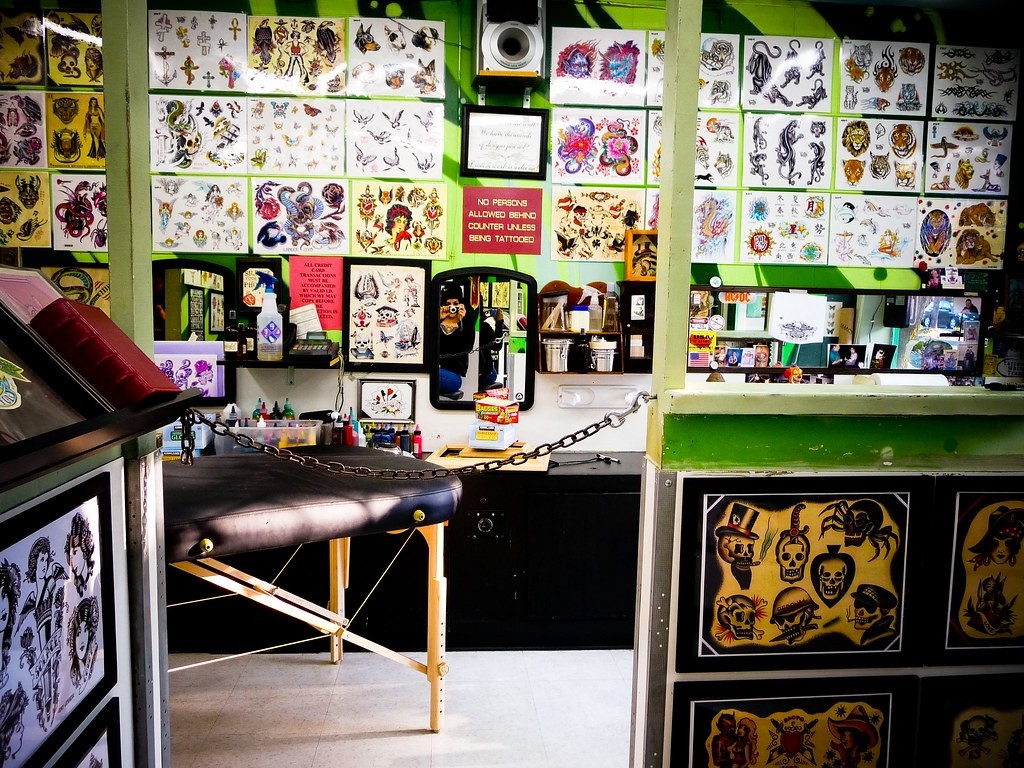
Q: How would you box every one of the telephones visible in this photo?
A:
[289,332,339,362]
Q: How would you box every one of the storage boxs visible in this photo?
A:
[469,397,520,451]
[164,413,215,458]
[213,417,323,454]
[473,388,509,402]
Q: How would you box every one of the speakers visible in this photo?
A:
[471,0,546,83]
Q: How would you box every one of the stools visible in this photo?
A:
[164,445,465,735]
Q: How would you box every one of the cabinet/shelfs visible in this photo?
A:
[536,280,624,375]
[171,451,647,653]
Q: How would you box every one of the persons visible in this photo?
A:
[843,346,859,365]
[964,344,976,368]
[830,345,842,365]
[962,299,979,314]
[949,316,957,329]
[439,284,474,399]
[714,347,726,365]
[728,351,739,367]
[755,346,767,367]
[923,314,931,327]
[478,292,504,390]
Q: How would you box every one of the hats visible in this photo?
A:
[440,283,464,305]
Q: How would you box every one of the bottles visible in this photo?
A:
[219,397,321,456]
[331,407,423,461]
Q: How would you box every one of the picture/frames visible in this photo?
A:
[686,284,989,378]
[0,472,118,768]
[341,257,432,373]
[0,264,118,462]
[51,696,123,768]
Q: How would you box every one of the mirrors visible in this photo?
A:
[430,267,537,411]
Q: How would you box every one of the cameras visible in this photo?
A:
[447,306,460,314]
[490,309,497,317]
